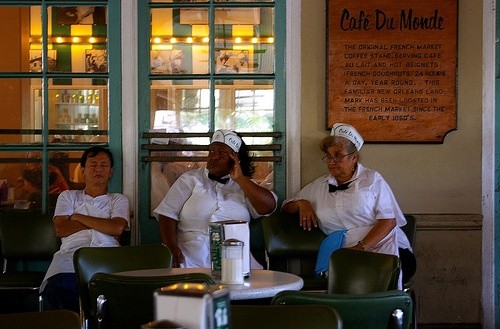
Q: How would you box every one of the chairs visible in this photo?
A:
[0,214,418,329]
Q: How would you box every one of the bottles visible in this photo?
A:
[221,239,244,286]
[49,90,101,143]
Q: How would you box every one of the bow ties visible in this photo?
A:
[328,181,352,193]
[208,173,230,185]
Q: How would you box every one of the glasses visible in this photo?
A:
[322,153,353,161]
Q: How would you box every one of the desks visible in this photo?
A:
[110,266,303,301]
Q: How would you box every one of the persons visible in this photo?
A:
[281,122,417,329]
[12,151,69,209]
[39,147,130,329]
[152,129,278,271]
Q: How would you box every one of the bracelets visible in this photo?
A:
[358,240,367,251]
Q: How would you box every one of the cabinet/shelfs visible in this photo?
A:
[53,89,108,138]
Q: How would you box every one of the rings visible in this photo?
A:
[303,218,306,220]
[239,161,240,163]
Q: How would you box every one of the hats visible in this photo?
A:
[330,123,364,152]
[211,129,242,152]
